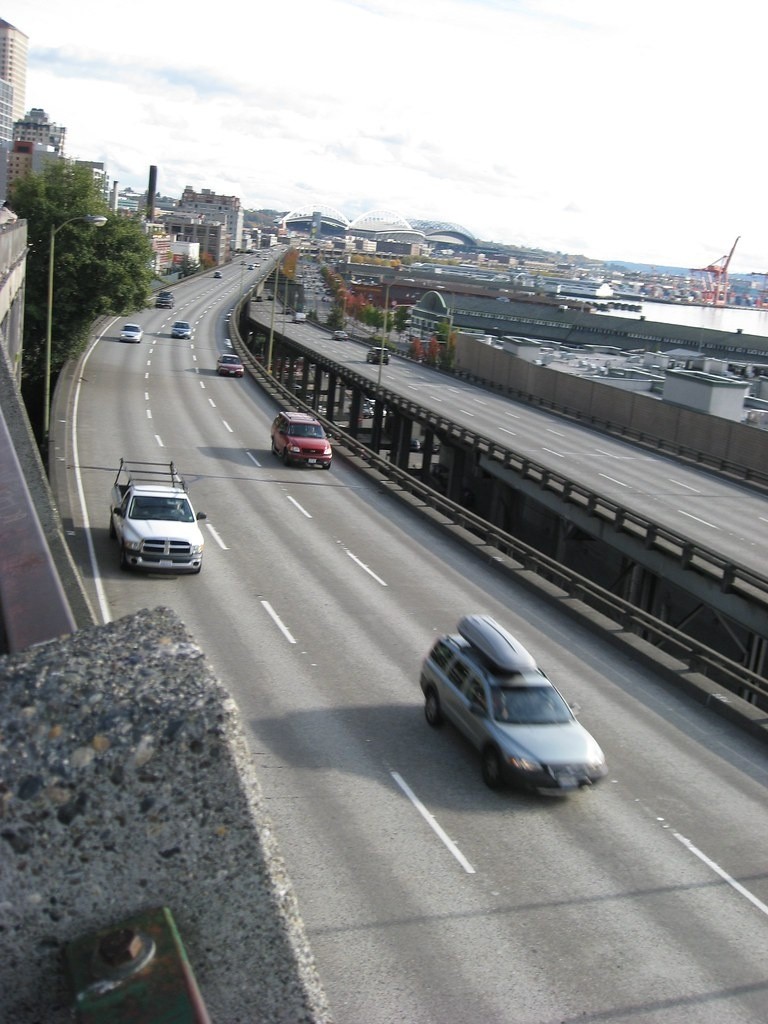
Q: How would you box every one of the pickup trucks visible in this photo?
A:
[107,458,208,575]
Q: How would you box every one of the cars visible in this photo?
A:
[216,354,245,378]
[213,271,222,278]
[332,330,348,342]
[234,245,332,303]
[119,323,144,343]
[170,320,192,340]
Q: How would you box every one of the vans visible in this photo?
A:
[292,312,307,323]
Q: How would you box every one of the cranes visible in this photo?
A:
[689,235,742,305]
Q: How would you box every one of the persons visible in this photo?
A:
[171,504,185,515]
[307,427,316,434]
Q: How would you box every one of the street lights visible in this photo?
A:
[376,277,415,383]
[40,215,109,446]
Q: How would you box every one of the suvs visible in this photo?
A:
[155,291,176,309]
[366,347,390,365]
[419,613,610,799]
[270,410,333,471]
